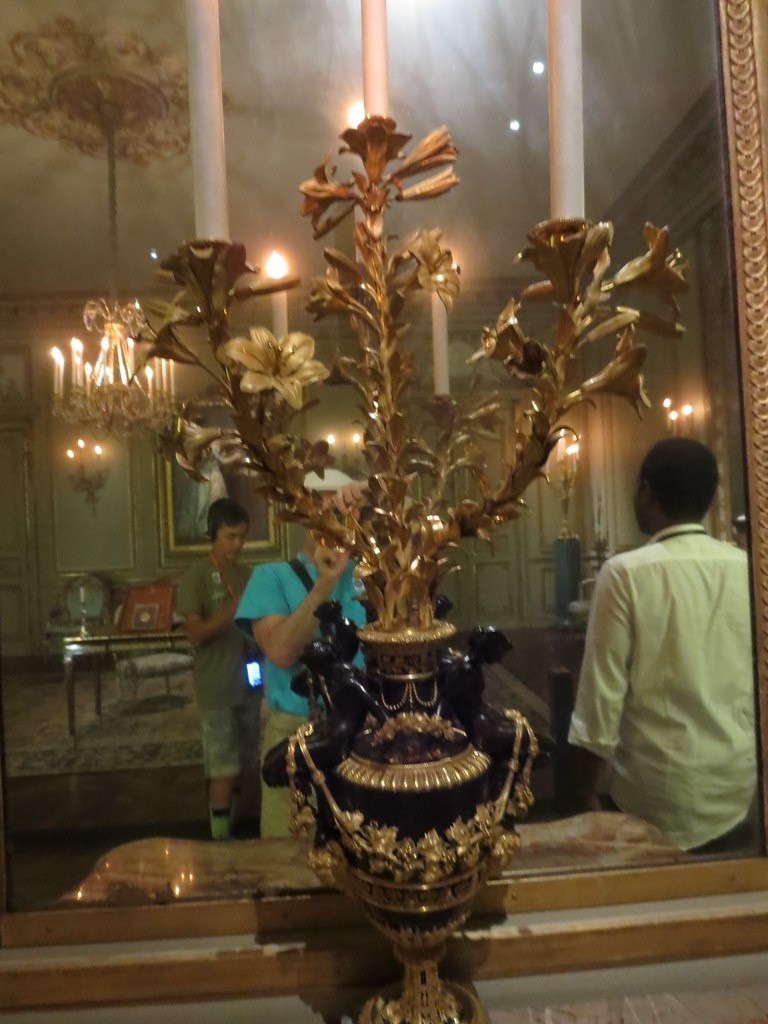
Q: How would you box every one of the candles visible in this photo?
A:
[428,272,454,395]
[50,327,175,404]
[266,251,290,343]
[544,0,585,223]
[361,0,391,120]
[186,1,227,240]
[660,394,700,439]
[557,429,580,474]
[79,586,86,610]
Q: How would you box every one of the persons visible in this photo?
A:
[176,498,263,842]
[235,469,368,840]
[567,437,762,856]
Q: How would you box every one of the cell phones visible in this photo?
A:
[331,509,348,554]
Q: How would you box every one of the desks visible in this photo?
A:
[62,627,198,738]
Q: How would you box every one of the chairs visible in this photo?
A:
[111,604,195,706]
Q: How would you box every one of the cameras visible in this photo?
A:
[244,659,264,690]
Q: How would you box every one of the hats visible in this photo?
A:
[303,469,353,493]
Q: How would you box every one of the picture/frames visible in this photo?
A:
[153,401,293,572]
